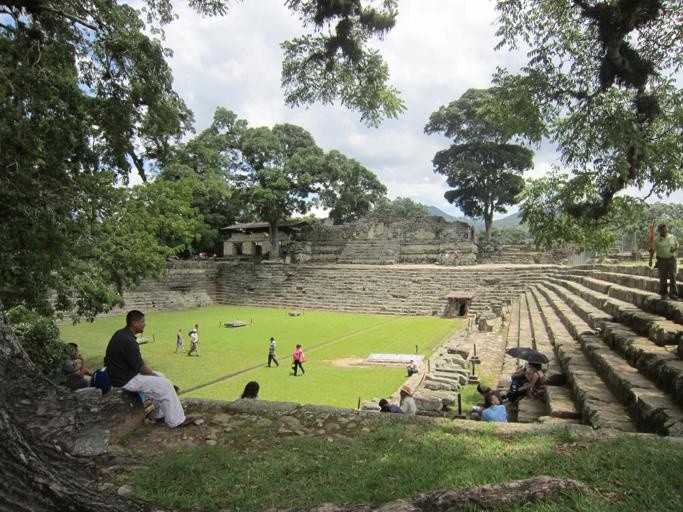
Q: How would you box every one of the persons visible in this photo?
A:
[90,355,114,396]
[175,329,185,354]
[104,309,202,427]
[59,341,96,389]
[405,359,419,376]
[265,337,279,367]
[292,344,305,376]
[646,223,680,301]
[193,323,199,332]
[399,384,417,415]
[187,329,199,356]
[240,382,260,400]
[151,384,181,423]
[467,359,547,423]
[378,398,402,414]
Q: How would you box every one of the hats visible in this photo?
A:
[400,384,414,396]
[658,223,665,229]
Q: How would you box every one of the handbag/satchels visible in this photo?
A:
[90,366,111,395]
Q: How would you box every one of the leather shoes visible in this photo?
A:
[179,415,197,426]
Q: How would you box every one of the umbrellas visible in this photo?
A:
[504,346,550,365]
